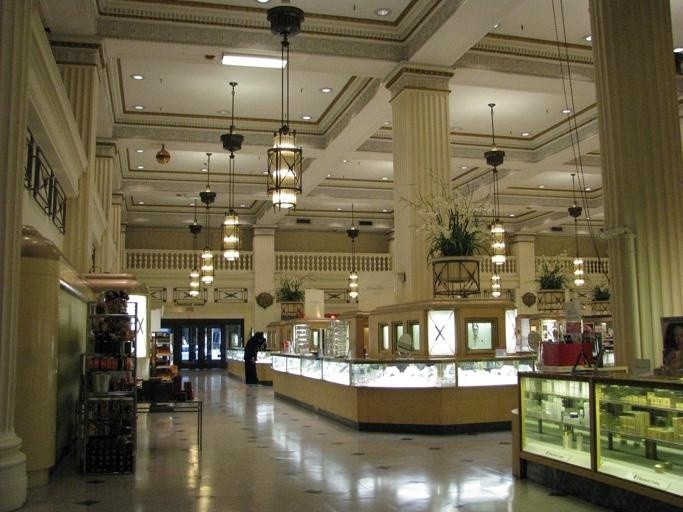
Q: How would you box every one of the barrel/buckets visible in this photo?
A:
[90,370,110,392]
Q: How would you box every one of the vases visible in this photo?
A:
[442,237,471,256]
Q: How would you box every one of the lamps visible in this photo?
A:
[486,104,508,267]
[344,203,361,299]
[490,269,504,298]
[264,2,306,215]
[567,172,587,288]
[218,77,245,265]
[186,222,202,299]
[196,152,215,287]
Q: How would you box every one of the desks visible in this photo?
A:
[133,401,202,453]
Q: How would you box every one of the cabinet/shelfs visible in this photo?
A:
[271,351,536,434]
[71,300,138,474]
[514,371,682,512]
[150,329,176,381]
[224,346,277,384]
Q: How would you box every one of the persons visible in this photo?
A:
[258,336,265,348]
[663,323,682,350]
[243,332,259,383]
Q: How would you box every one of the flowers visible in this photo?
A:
[534,250,576,289]
[398,171,501,257]
[587,270,611,300]
[274,268,315,302]
